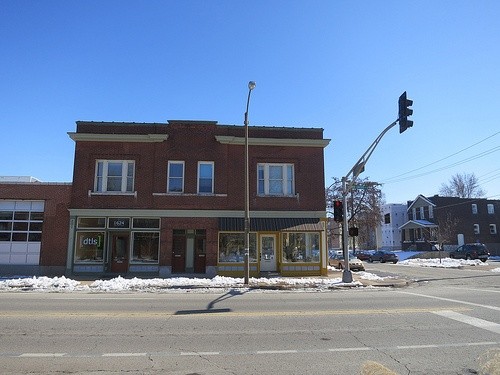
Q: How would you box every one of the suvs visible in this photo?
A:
[450,243,490,262]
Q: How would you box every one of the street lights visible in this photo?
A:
[244,81,257,284]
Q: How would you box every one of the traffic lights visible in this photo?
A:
[398,91,413,134]
[334,201,343,222]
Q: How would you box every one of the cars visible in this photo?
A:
[313,250,399,271]
